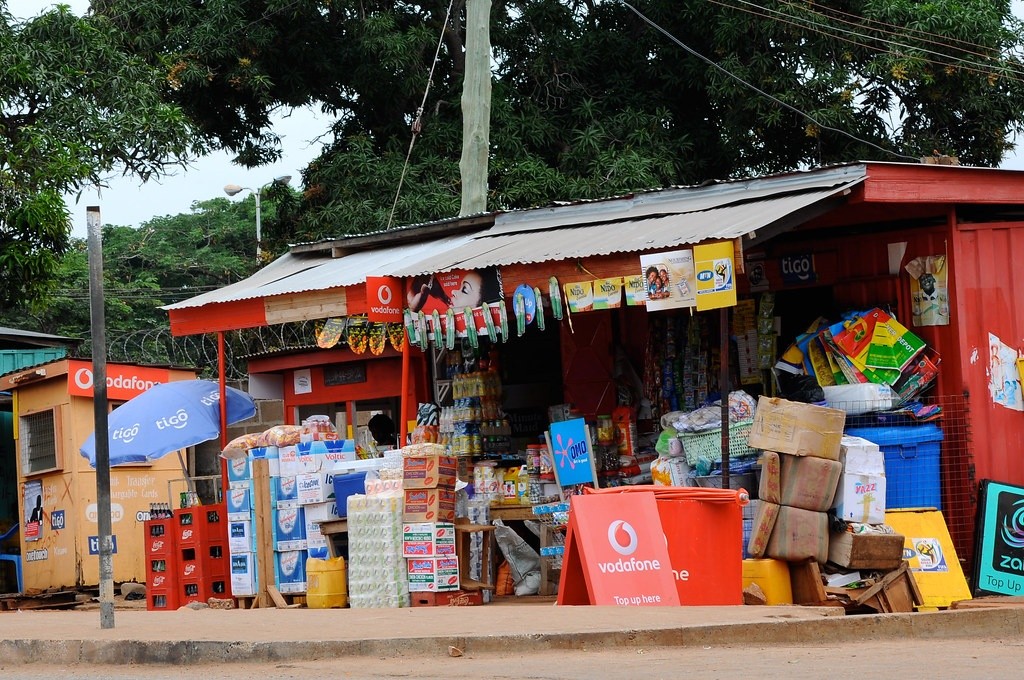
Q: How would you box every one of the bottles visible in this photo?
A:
[146,500,172,606]
[179,492,199,598]
[207,489,225,595]
[416,274,451,306]
[411,345,514,455]
[300,420,332,441]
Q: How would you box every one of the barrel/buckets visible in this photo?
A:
[305,556,347,608]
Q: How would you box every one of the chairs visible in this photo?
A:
[0,523,23,593]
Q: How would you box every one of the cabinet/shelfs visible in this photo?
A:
[431,341,466,407]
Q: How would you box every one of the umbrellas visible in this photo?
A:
[79,380,257,492]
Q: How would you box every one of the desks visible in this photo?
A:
[312,519,348,559]
[489,505,562,596]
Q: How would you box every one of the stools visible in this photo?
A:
[454,518,497,590]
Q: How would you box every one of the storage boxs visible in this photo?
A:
[332,457,385,518]
[143,503,231,611]
[225,439,356,596]
[746,383,945,570]
[402,455,483,606]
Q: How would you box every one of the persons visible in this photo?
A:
[646,267,670,301]
[406,268,501,315]
[368,413,397,448]
